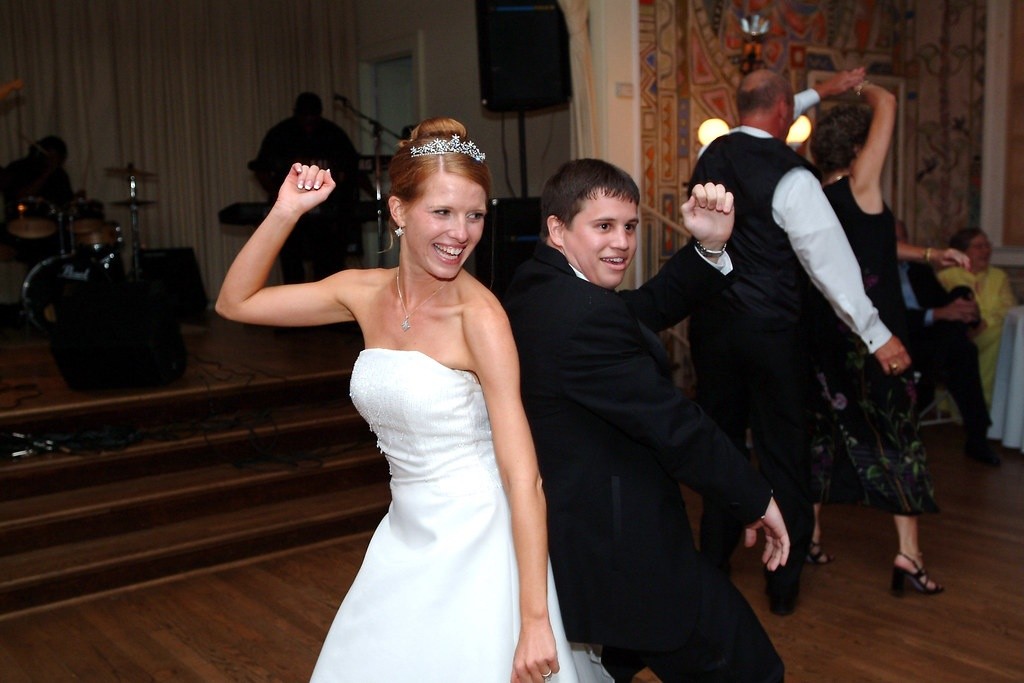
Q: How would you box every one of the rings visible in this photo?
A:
[542,670,551,677]
[891,364,899,372]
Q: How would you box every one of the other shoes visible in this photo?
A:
[769,593,794,615]
[964,440,1000,465]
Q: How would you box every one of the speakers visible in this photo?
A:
[476,197,543,299]
[132,247,206,320]
[474,0,572,113]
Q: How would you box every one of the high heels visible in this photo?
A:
[804,540,836,564]
[891,552,945,598]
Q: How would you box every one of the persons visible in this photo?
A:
[212,112,613,682]
[255,93,358,285]
[895,221,1016,464]
[1,135,83,329]
[690,69,945,616]
[504,157,786,683]
[809,79,943,596]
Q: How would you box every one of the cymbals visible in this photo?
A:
[103,167,158,180]
[108,199,160,207]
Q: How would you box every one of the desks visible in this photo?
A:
[987,306,1024,452]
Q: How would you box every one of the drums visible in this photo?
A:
[3,195,62,240]
[62,197,105,234]
[73,219,122,247]
[17,255,114,337]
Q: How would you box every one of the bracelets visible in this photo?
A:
[925,247,931,262]
[857,79,871,96]
[697,240,726,257]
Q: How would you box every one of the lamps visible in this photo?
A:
[741,14,770,73]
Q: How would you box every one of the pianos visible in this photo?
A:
[215,194,392,284]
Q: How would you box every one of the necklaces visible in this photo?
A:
[833,175,842,182]
[396,266,447,331]
